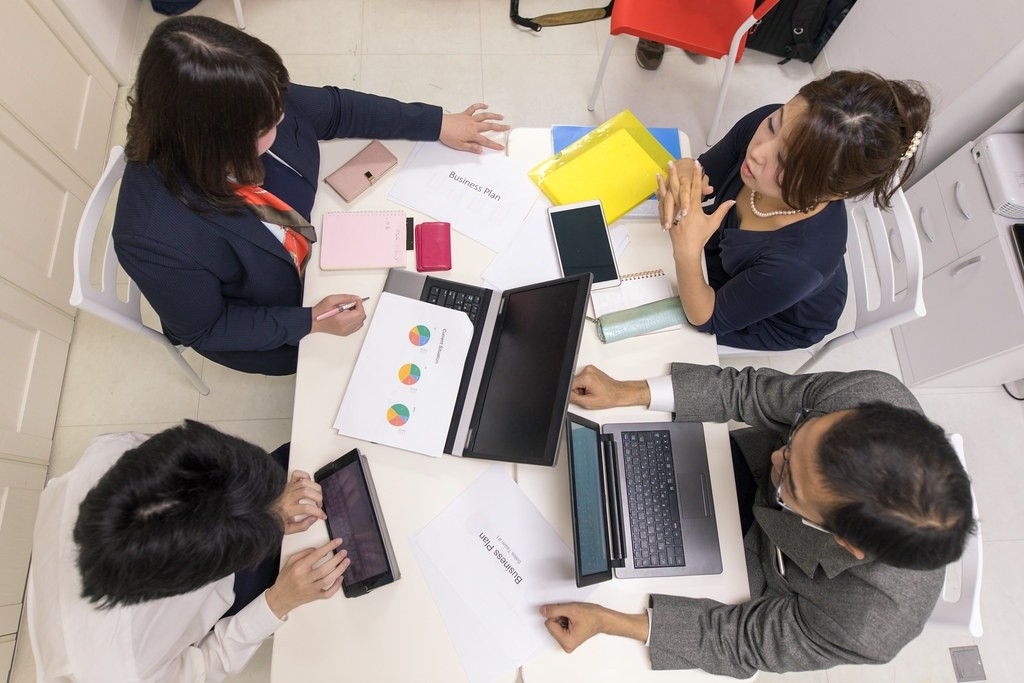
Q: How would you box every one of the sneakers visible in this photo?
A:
[635,36,666,71]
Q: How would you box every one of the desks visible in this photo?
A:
[269,128,764,683]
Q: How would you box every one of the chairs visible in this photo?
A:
[587,0,781,146]
[719,166,927,375]
[70,145,210,396]
[931,437,987,639]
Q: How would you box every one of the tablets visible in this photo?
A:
[547,199,622,291]
[314,447,394,598]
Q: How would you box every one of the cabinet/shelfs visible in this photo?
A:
[882,103,1024,391]
[0,0,118,683]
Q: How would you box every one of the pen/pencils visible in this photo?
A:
[672,165,707,226]
[315,295,371,322]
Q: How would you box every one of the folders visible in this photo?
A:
[550,124,681,219]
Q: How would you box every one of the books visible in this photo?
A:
[590,269,682,336]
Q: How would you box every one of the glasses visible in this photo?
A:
[773,407,834,538]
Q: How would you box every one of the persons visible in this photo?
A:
[112,15,511,376]
[654,70,931,351]
[26,418,350,683]
[539,362,973,679]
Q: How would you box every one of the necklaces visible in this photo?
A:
[749,189,814,218]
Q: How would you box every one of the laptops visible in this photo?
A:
[380,267,594,468]
[565,411,724,589]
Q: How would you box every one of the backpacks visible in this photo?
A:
[745,0,859,66]
